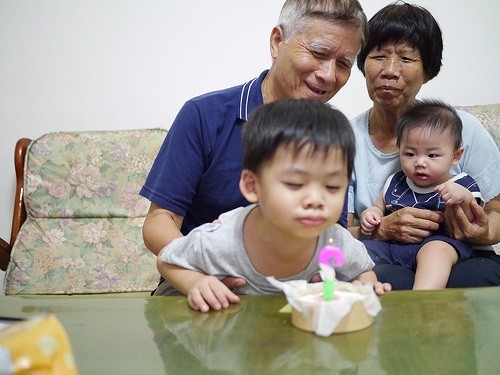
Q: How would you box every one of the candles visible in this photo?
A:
[320,238,343,300]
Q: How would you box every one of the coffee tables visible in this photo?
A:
[0,286,500,375]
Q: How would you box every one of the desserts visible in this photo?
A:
[292,246,364,304]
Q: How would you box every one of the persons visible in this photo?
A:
[157,96,392,313]
[347,0,500,291]
[139,0,370,296]
[360,99,486,290]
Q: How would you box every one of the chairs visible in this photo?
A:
[0,128,169,295]
[452,103,500,257]
